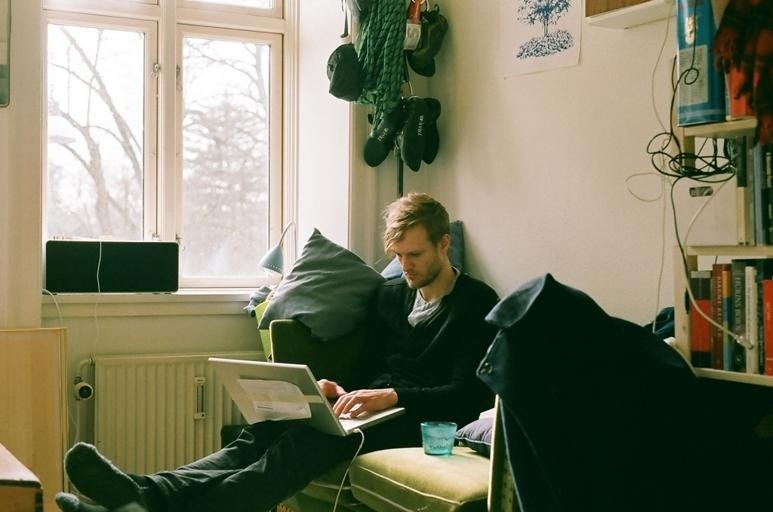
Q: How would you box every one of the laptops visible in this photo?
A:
[207,357,406,438]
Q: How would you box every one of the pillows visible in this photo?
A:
[453,416,493,457]
[381,220,465,278]
[258,227,386,343]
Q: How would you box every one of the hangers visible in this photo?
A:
[360,0,439,198]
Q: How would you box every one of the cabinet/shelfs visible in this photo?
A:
[676,0,772,389]
[583,1,680,29]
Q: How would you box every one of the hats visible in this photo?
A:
[407,9,449,77]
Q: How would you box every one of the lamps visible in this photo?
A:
[259,221,298,279]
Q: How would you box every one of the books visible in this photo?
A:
[765,150,773,245]
[762,278,773,376]
[738,137,747,247]
[746,264,758,373]
[712,263,716,367]
[716,264,732,369]
[746,137,757,247]
[758,260,765,374]
[722,270,732,369]
[691,270,713,368]
[677,0,725,126]
[733,259,747,372]
[725,80,745,123]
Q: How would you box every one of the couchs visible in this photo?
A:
[283,445,494,511]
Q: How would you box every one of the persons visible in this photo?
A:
[55,194,503,512]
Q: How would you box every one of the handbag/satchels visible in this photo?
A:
[326,43,362,101]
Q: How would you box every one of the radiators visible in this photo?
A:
[93,351,267,475]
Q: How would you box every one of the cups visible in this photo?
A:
[419,421,456,456]
